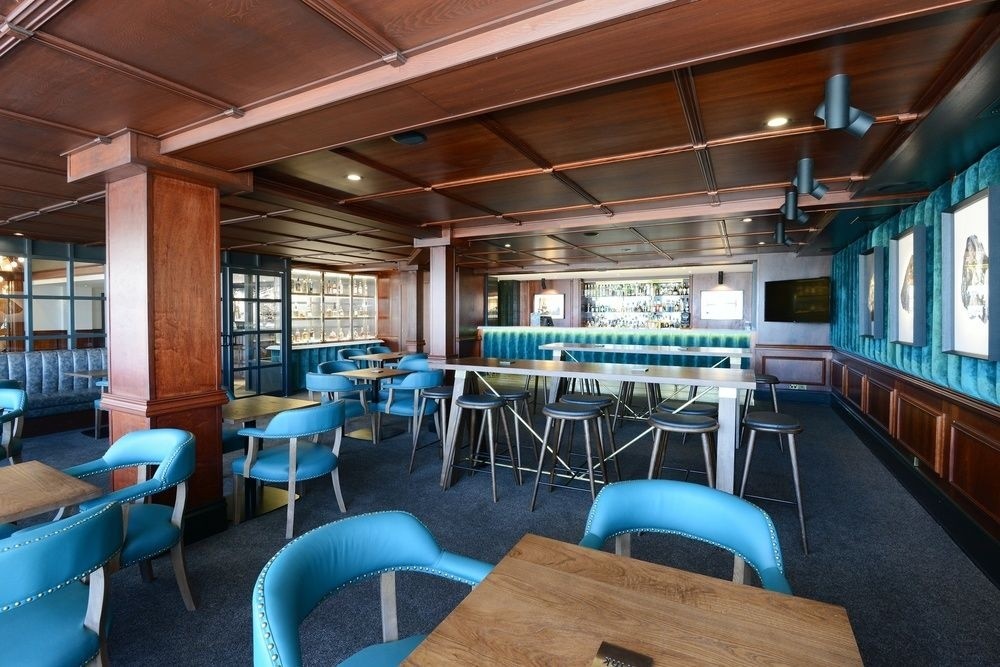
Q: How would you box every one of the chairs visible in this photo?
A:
[573,475,794,596]
[367,345,397,370]
[228,400,351,539]
[371,352,445,443]
[303,371,381,445]
[338,345,370,375]
[47,425,199,612]
[2,386,28,459]
[250,504,493,666]
[316,360,369,418]
[1,495,128,667]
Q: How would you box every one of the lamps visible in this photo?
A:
[767,67,879,248]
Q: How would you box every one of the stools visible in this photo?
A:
[406,352,817,561]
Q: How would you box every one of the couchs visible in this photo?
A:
[2,348,109,420]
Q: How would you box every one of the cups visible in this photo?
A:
[744,322,751,331]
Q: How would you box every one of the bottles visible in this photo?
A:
[584,281,690,329]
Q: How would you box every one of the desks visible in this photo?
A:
[330,365,417,442]
[219,393,321,527]
[535,341,743,449]
[1,457,104,522]
[399,531,864,667]
